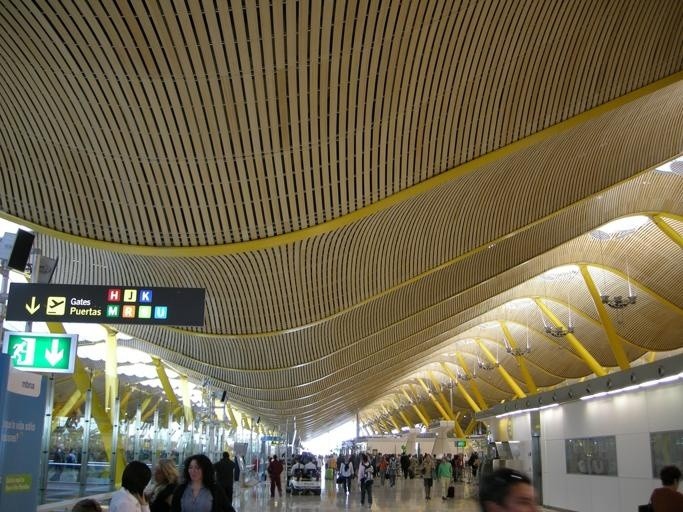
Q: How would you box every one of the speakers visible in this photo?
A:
[254,416,260,423]
[213,391,227,402]
[0,228,36,272]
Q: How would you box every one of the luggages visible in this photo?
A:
[448,487,455,498]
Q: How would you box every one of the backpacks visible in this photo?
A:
[362,462,374,485]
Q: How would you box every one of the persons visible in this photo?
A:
[212,452,236,506]
[72,499,101,512]
[649,465,683,512]
[477,466,539,512]
[49,443,96,481]
[267,454,283,498]
[108,461,152,512]
[172,454,236,512]
[147,458,181,512]
[289,451,479,512]
